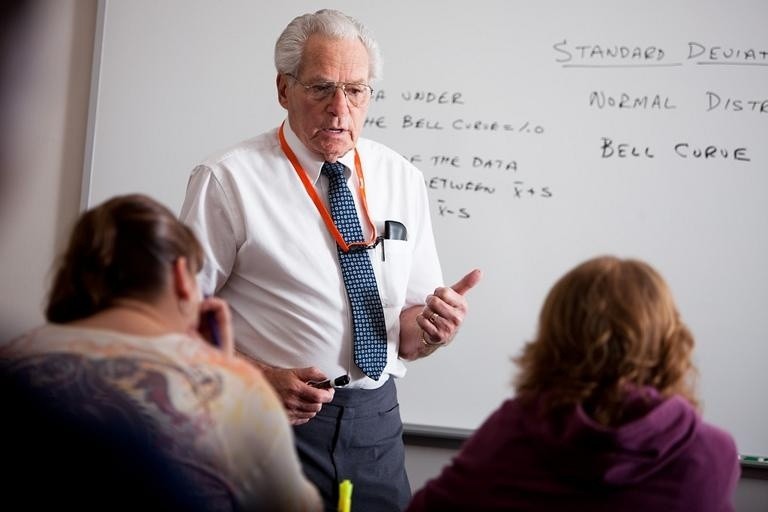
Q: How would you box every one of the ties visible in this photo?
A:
[321,160,388,380]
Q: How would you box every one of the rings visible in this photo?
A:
[428,313,439,323]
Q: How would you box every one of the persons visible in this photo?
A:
[399,252,745,510]
[0,190,331,512]
[177,5,483,510]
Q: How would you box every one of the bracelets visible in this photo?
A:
[419,330,444,349]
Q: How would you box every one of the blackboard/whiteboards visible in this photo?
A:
[81,0,768,475]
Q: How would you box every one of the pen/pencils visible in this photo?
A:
[313,375,350,389]
[338,478,353,512]
[201,292,219,348]
[736,454,768,463]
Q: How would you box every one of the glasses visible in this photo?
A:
[286,73,374,108]
